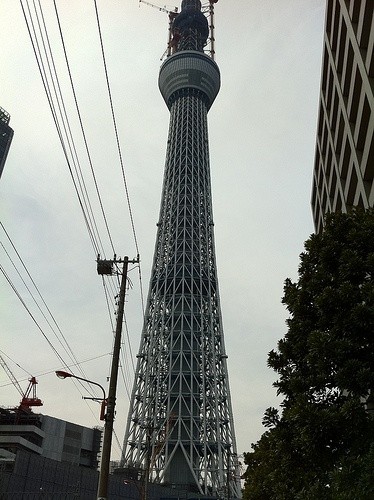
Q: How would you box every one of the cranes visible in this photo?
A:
[0,350,43,427]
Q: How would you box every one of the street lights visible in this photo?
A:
[54,368,116,499]
